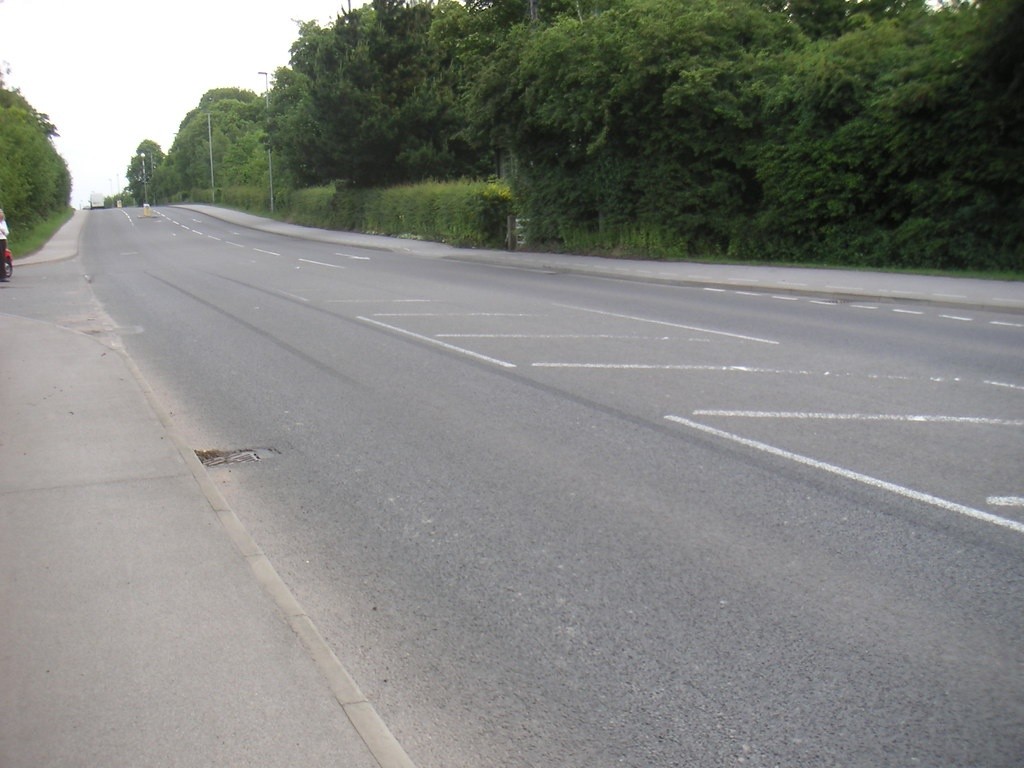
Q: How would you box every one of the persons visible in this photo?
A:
[0,209,9,282]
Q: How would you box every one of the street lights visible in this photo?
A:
[202,113,216,205]
[258,72,275,214]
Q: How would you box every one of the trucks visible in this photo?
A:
[88,192,106,210]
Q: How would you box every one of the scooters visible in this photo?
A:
[3,247,13,276]
